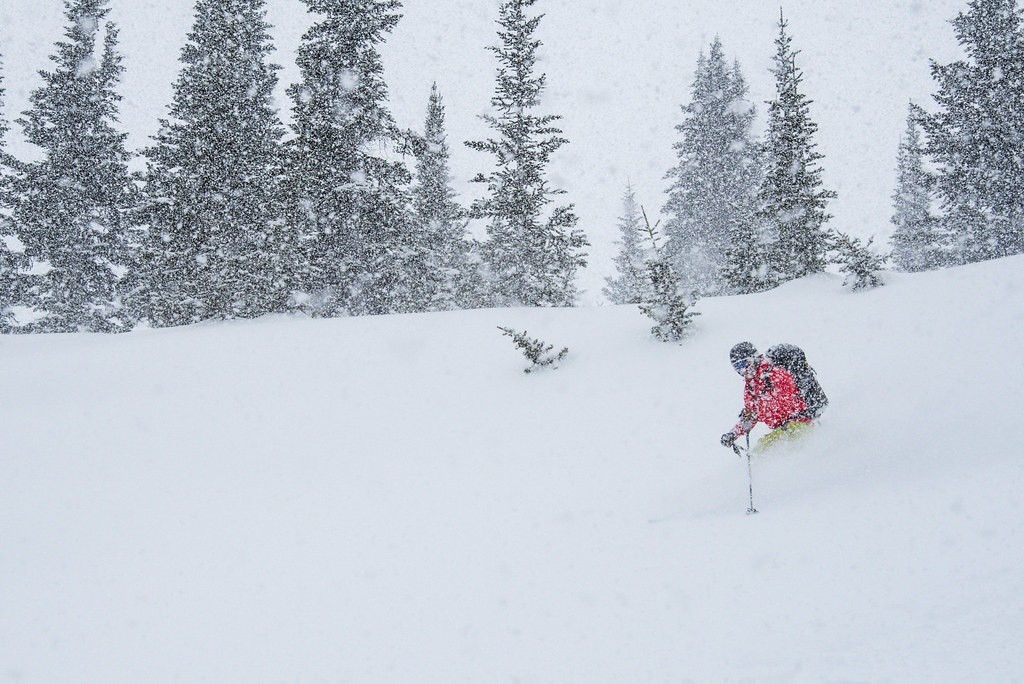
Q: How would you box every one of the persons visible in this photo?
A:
[722,342,811,459]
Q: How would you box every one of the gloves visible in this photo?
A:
[721,433,735,448]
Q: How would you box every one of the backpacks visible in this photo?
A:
[766,343,828,429]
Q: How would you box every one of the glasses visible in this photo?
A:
[732,351,763,370]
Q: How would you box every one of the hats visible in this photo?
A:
[730,341,757,364]
